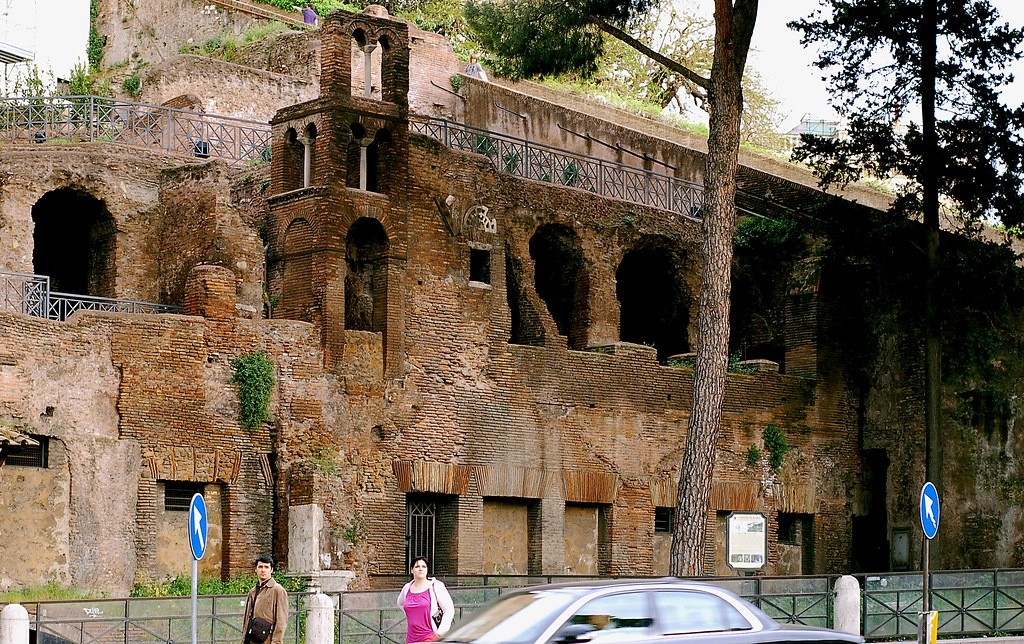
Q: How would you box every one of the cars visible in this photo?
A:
[435,575,867,644]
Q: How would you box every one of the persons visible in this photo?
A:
[397,556,454,644]
[464,56,486,81]
[241,554,289,644]
[588,615,616,630]
[292,3,318,27]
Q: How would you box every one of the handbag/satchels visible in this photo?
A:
[246,617,275,644]
[432,583,457,633]
